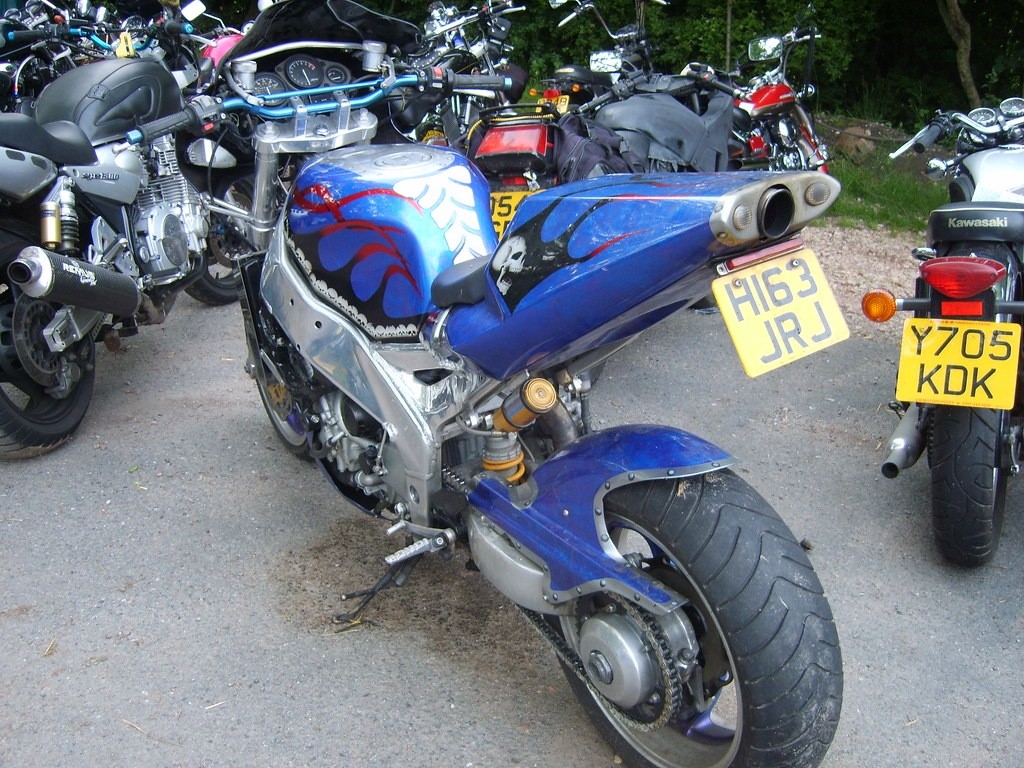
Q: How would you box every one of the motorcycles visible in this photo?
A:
[861,97,1024,565]
[0,0,830,457]
[127,0,844,768]
